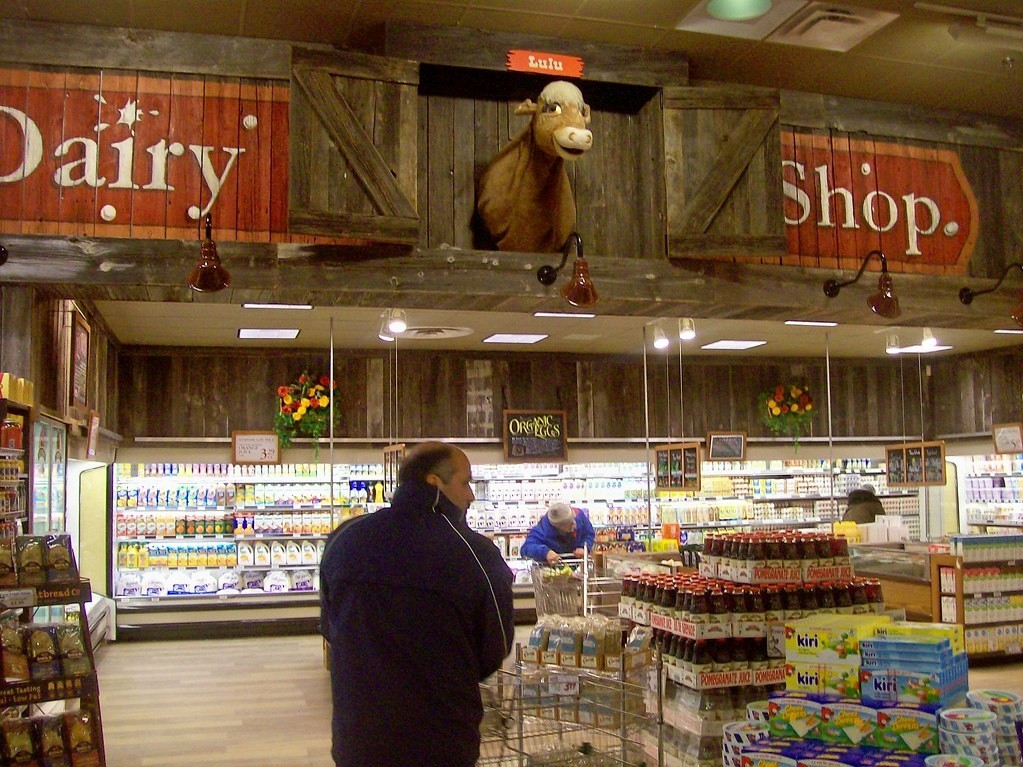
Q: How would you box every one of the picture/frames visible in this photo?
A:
[69,310,91,409]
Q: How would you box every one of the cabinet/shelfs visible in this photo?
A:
[0,446,28,524]
[930,553,1023,666]
[0,577,106,767]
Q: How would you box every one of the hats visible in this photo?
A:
[547,502,574,527]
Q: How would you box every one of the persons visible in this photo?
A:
[520,502,596,571]
[843,485,885,524]
[319,441,514,767]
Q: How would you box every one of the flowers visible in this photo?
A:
[756,378,817,455]
[273,371,343,463]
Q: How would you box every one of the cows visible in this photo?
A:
[476,80,594,253]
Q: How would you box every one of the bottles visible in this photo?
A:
[118,543,147,567]
[238,540,327,566]
[0,418,26,551]
[621,530,885,767]
[351,480,384,502]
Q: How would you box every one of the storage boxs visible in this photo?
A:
[0,372,34,407]
[740,614,969,767]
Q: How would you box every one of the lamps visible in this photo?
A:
[188,213,231,292]
[678,317,695,340]
[921,327,937,348]
[378,307,406,341]
[823,249,902,320]
[652,322,669,349]
[960,262,1023,326]
[885,329,899,354]
[537,232,600,307]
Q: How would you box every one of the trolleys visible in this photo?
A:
[531,541,596,619]
[472,639,665,767]
